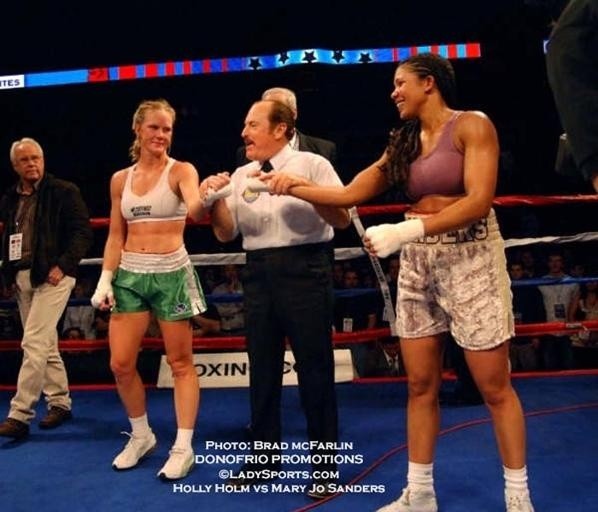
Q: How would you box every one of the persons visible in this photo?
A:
[199,100,354,501]
[234,86,342,435]
[545,3,597,194]
[89,99,230,481]
[246,52,534,510]
[0,137,92,439]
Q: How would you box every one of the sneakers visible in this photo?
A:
[0,416,31,439]
[222,462,281,491]
[306,481,338,499]
[503,488,534,512]
[156,444,197,480]
[377,483,438,512]
[39,405,73,430]
[111,427,159,471]
